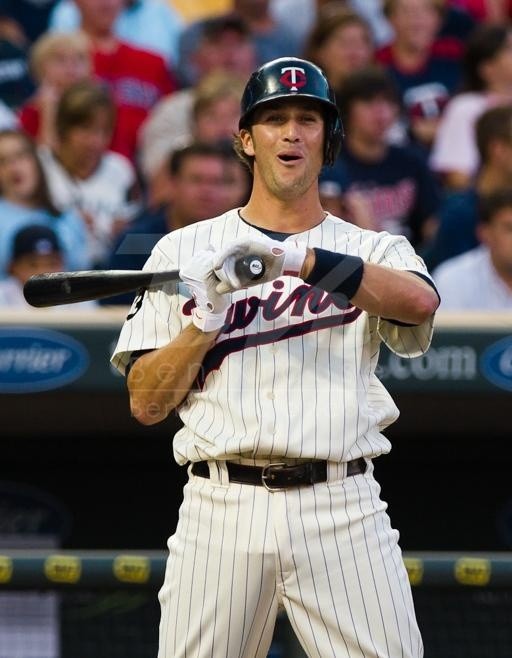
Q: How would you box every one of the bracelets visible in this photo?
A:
[298,242,366,300]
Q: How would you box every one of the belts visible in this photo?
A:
[191,457,367,491]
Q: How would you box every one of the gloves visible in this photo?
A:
[179,245,228,333]
[212,235,307,294]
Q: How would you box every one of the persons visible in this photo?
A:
[2,1,512,329]
[112,55,445,658]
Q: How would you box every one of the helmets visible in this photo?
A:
[239,57,342,142]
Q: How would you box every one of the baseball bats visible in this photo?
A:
[23,254,266,307]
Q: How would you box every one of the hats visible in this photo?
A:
[403,82,450,120]
[14,225,59,261]
